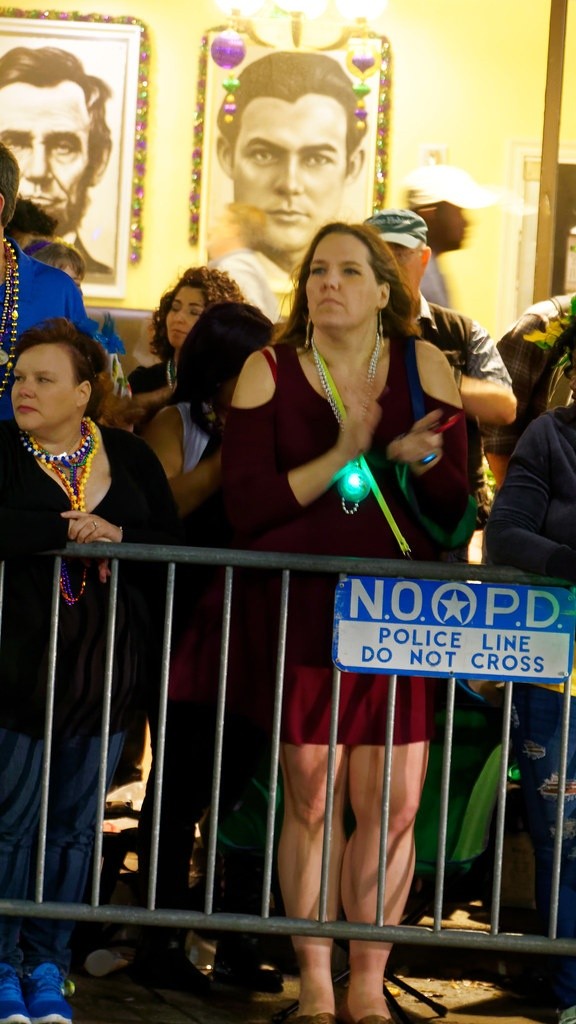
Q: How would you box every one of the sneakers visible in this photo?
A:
[0,963,31,1024]
[23,962,72,1024]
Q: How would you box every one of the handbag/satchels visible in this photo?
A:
[410,699,515,876]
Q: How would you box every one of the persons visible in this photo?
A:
[201,51,367,325]
[0,46,114,278]
[0,263,284,1024]
[220,210,576,1024]
[406,166,484,310]
[0,142,132,459]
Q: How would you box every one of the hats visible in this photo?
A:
[364,209,427,248]
[399,165,498,210]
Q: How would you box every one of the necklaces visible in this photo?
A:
[0,236,21,404]
[17,417,102,608]
[311,332,381,513]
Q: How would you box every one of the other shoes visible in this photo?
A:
[290,1001,337,1024]
[340,989,395,1024]
[130,950,215,992]
[481,969,573,1008]
[212,956,283,991]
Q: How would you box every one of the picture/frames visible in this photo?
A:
[0,18,142,299]
[198,28,394,316]
